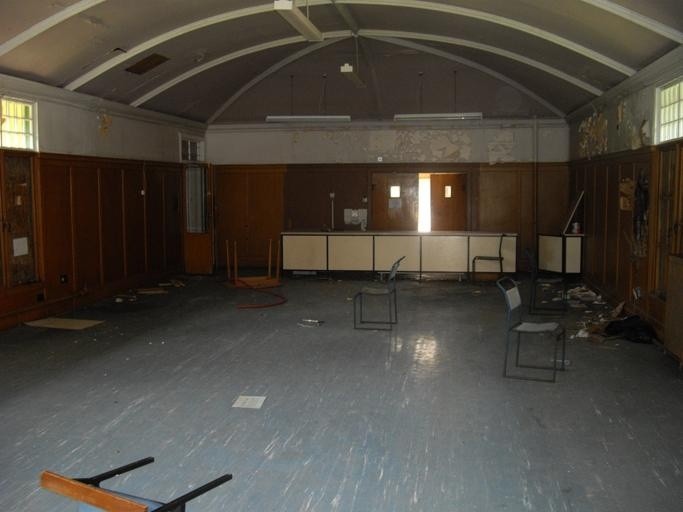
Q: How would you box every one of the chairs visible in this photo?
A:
[491,271,574,385]
[472,232,506,286]
[349,255,405,335]
[40,455,237,511]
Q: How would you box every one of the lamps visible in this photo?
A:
[273,1,329,45]
[338,31,368,92]
[262,74,353,126]
[391,68,486,124]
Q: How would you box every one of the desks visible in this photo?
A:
[223,235,283,292]
